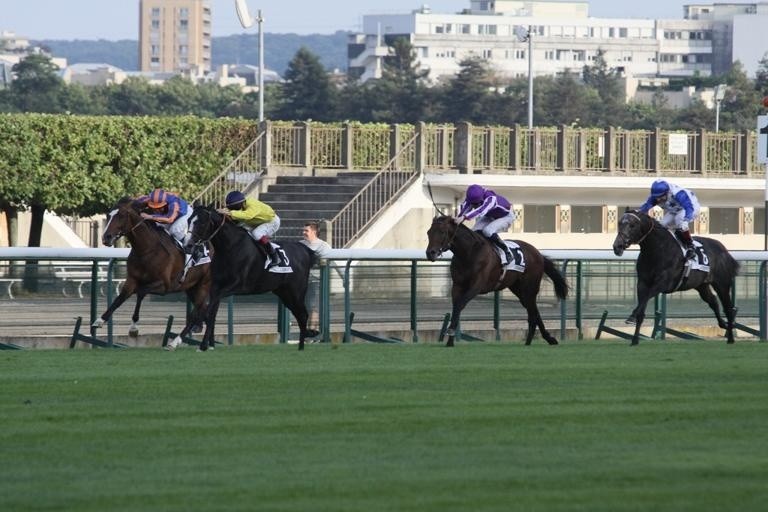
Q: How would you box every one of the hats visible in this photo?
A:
[146,184,168,209]
[651,180,669,196]
[225,190,244,209]
[466,185,483,203]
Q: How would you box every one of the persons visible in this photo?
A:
[453,184,518,266]
[136,188,208,284]
[215,190,283,268]
[636,180,701,261]
[298,222,333,342]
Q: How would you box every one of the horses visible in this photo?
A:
[91,195,217,352]
[425,213,571,348]
[165,199,323,352]
[612,205,742,347]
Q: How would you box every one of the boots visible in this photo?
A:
[494,237,507,250]
[264,243,283,268]
[686,244,696,258]
[189,242,204,261]
